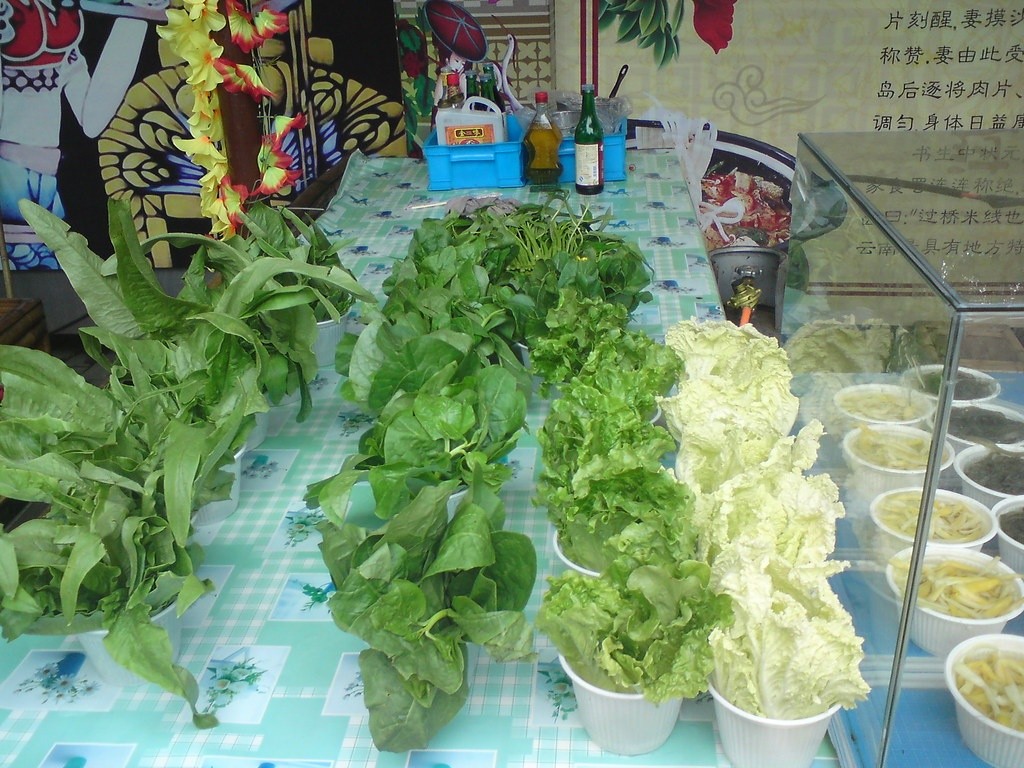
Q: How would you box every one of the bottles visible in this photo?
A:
[519,91,563,193]
[431,61,504,144]
[574,84,604,194]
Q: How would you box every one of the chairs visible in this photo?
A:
[0,216,51,356]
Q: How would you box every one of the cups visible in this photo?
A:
[192,445,245,524]
[263,385,308,405]
[347,337,846,768]
[228,400,271,450]
[310,306,354,368]
[76,602,183,689]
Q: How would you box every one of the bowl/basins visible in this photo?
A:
[870,487,998,557]
[954,444,1024,510]
[943,632,1024,768]
[834,385,935,434]
[925,402,1024,453]
[992,494,1024,574]
[550,111,582,137]
[901,365,1001,407]
[627,118,848,253]
[886,549,1024,656]
[842,424,955,490]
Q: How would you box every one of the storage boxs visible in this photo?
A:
[916,320,1024,372]
[420,114,628,192]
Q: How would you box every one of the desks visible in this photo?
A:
[0,151,839,768]
[777,371,1024,768]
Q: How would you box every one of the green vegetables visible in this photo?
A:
[1,195,870,755]
